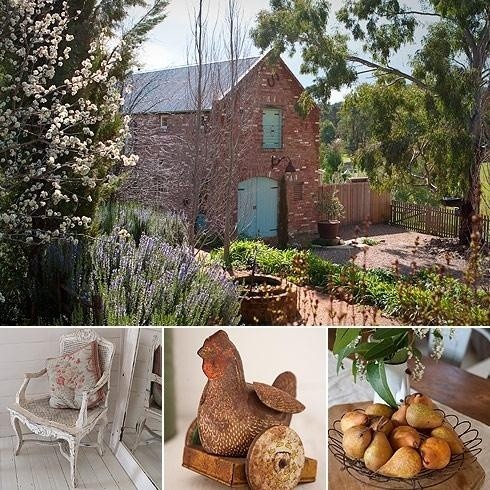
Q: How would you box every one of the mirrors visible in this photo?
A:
[108,329,162,490]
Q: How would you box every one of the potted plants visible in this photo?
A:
[315,188,345,238]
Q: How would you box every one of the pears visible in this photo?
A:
[340,391,464,479]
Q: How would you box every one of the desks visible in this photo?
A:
[329,350,489,490]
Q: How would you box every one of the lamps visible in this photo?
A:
[269,155,296,173]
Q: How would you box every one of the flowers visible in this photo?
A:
[332,328,457,408]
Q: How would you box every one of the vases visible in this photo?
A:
[372,362,410,404]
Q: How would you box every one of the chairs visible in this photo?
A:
[8,331,116,488]
[129,341,163,455]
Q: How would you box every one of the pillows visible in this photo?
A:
[47,340,103,409]
